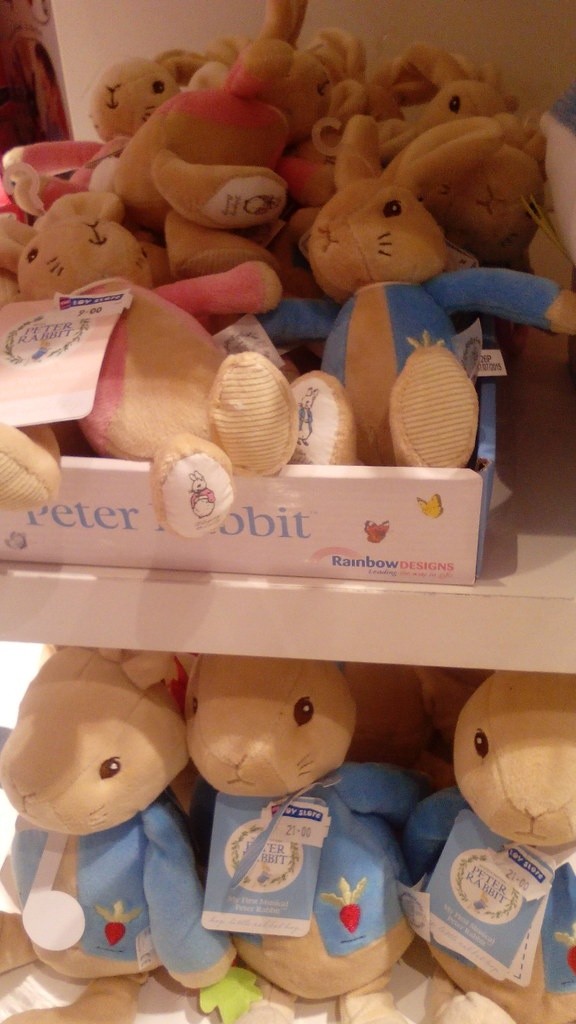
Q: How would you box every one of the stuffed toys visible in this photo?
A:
[0,647,575,1024]
[0,1,576,539]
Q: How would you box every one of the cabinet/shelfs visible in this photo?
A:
[0,1,576,1023]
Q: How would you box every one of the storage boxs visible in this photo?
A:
[0,320,497,587]
